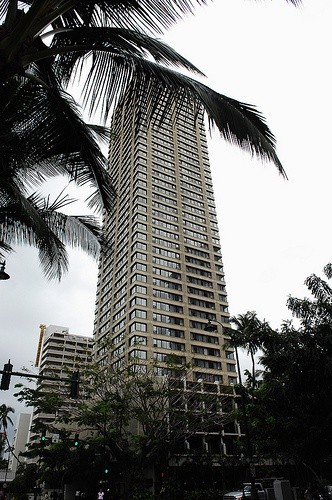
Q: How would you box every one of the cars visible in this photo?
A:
[223,478,287,500]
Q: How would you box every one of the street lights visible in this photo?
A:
[2,481,8,500]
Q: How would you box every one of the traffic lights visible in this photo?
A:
[96,465,112,476]
[74,434,79,446]
[42,429,46,441]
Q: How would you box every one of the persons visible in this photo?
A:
[0,487,110,500]
